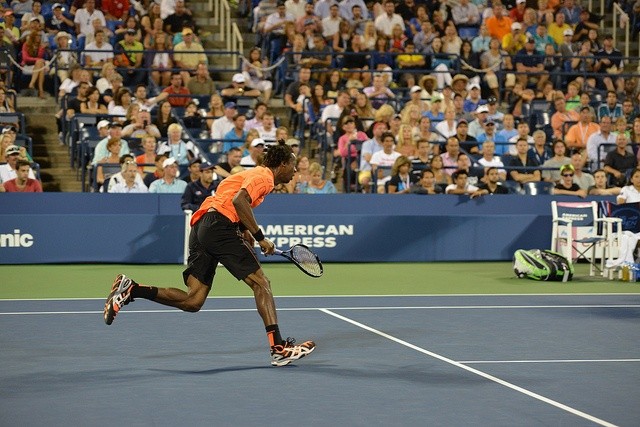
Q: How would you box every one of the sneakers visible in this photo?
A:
[104,273,139,325]
[270,337,317,367]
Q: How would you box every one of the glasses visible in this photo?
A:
[485,124,497,127]
[562,174,573,177]
[123,161,135,166]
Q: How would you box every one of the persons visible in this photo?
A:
[509,120,535,155]
[235,0,252,17]
[413,118,438,143]
[205,93,225,129]
[440,84,456,120]
[541,43,560,72]
[587,170,622,196]
[183,101,202,128]
[536,80,554,100]
[396,124,417,157]
[44,3,75,35]
[528,23,558,54]
[253,111,278,148]
[143,155,169,188]
[351,93,375,118]
[570,10,600,43]
[564,105,601,150]
[621,79,638,101]
[0,87,15,113]
[472,140,505,167]
[596,34,625,92]
[387,155,411,194]
[19,1,45,35]
[67,81,90,119]
[546,12,576,45]
[283,33,313,74]
[521,88,536,120]
[450,152,484,180]
[114,88,131,116]
[362,74,396,109]
[264,1,297,66]
[420,95,444,133]
[479,38,514,103]
[464,86,488,114]
[597,91,624,121]
[621,99,633,123]
[358,122,396,193]
[395,41,426,88]
[431,10,446,37]
[0,10,20,48]
[155,122,200,164]
[432,24,446,37]
[511,36,550,92]
[476,118,508,155]
[400,85,430,124]
[296,1,325,35]
[296,83,313,125]
[112,28,147,87]
[570,39,595,72]
[172,27,209,88]
[546,51,566,90]
[449,74,470,101]
[95,119,111,143]
[418,75,445,103]
[405,4,430,41]
[401,105,422,138]
[345,35,372,85]
[347,86,359,100]
[634,92,640,117]
[72,70,92,96]
[130,85,163,113]
[111,160,148,193]
[485,5,514,42]
[369,133,402,193]
[332,19,361,54]
[345,68,365,92]
[602,132,638,187]
[587,29,603,52]
[12,0,34,15]
[365,104,396,140]
[162,0,199,35]
[410,139,435,170]
[522,7,539,41]
[270,171,296,194]
[73,0,107,39]
[295,156,310,193]
[222,113,252,157]
[339,1,368,21]
[283,21,298,49]
[0,134,14,165]
[585,116,618,169]
[239,128,260,158]
[608,0,640,41]
[432,0,452,21]
[371,0,387,18]
[148,2,162,18]
[135,134,158,175]
[1,125,34,162]
[275,127,288,144]
[483,1,510,23]
[110,73,122,93]
[0,145,37,182]
[239,138,268,169]
[558,0,582,24]
[0,0,20,18]
[374,0,406,38]
[135,15,154,45]
[430,35,454,92]
[285,0,307,21]
[51,0,70,18]
[507,138,542,195]
[143,30,174,87]
[439,137,472,176]
[21,17,45,42]
[410,169,443,194]
[143,18,174,51]
[241,47,273,107]
[212,147,243,180]
[321,3,345,47]
[445,169,489,200]
[395,0,418,19]
[409,4,430,36]
[477,167,508,194]
[499,114,518,145]
[388,23,408,54]
[347,6,365,23]
[130,109,162,140]
[487,95,504,120]
[318,91,350,143]
[574,93,597,122]
[322,68,351,103]
[114,16,142,41]
[54,30,79,83]
[306,33,333,80]
[371,34,393,70]
[615,169,640,205]
[108,86,122,115]
[551,91,581,140]
[541,140,572,182]
[253,0,276,48]
[220,73,261,97]
[502,22,528,55]
[470,23,493,54]
[537,0,561,23]
[308,83,335,129]
[449,0,480,38]
[429,154,453,185]
[161,71,192,107]
[402,74,416,108]
[69,0,85,16]
[285,138,300,159]
[130,0,161,16]
[300,162,337,194]
[180,162,223,214]
[442,26,463,53]
[564,81,581,110]
[366,0,374,19]
[54,62,93,119]
[393,115,402,141]
[91,121,130,165]
[448,121,479,155]
[300,19,328,47]
[108,154,134,193]
[151,99,179,138]
[301,115,302,126]
[412,20,440,53]
[84,17,113,44]
[186,61,215,94]
[444,20,455,26]
[20,31,49,99]
[103,139,317,367]
[337,115,370,190]
[508,0,530,23]
[456,39,482,99]
[148,157,188,193]
[284,66,320,126]
[79,87,109,115]
[560,29,576,56]
[453,93,476,124]
[100,0,131,22]
[0,26,16,90]
[314,1,338,19]
[529,130,553,165]
[0,160,43,192]
[84,31,115,69]
[361,21,378,50]
[96,138,123,192]
[567,153,595,189]
[95,63,123,94]
[243,101,268,130]
[121,104,140,130]
[629,117,640,161]
[180,157,203,185]
[550,164,586,198]
[210,101,239,139]
[99,89,113,106]
[610,116,631,139]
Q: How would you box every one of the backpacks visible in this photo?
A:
[513,248,575,281]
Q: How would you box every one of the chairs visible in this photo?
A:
[551,200,615,281]
[598,199,640,280]
[1,1,122,62]
[61,82,251,192]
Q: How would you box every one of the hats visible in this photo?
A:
[563,29,574,36]
[250,138,265,146]
[182,27,193,36]
[54,31,72,43]
[525,37,536,43]
[511,22,521,30]
[224,102,239,110]
[482,118,497,127]
[475,105,490,114]
[456,119,468,126]
[96,119,111,130]
[451,74,469,90]
[162,159,179,169]
[199,161,215,170]
[431,96,442,105]
[5,145,21,157]
[560,164,575,173]
[580,104,590,111]
[286,139,301,146]
[51,3,62,10]
[411,85,422,93]
[29,17,40,23]
[419,75,438,91]
[189,158,202,165]
[232,73,246,83]
[2,125,16,134]
[110,122,124,127]
[341,116,354,124]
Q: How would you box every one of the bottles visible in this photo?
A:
[617,261,640,282]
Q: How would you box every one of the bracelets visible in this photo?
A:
[252,229,264,242]
[238,221,248,233]
